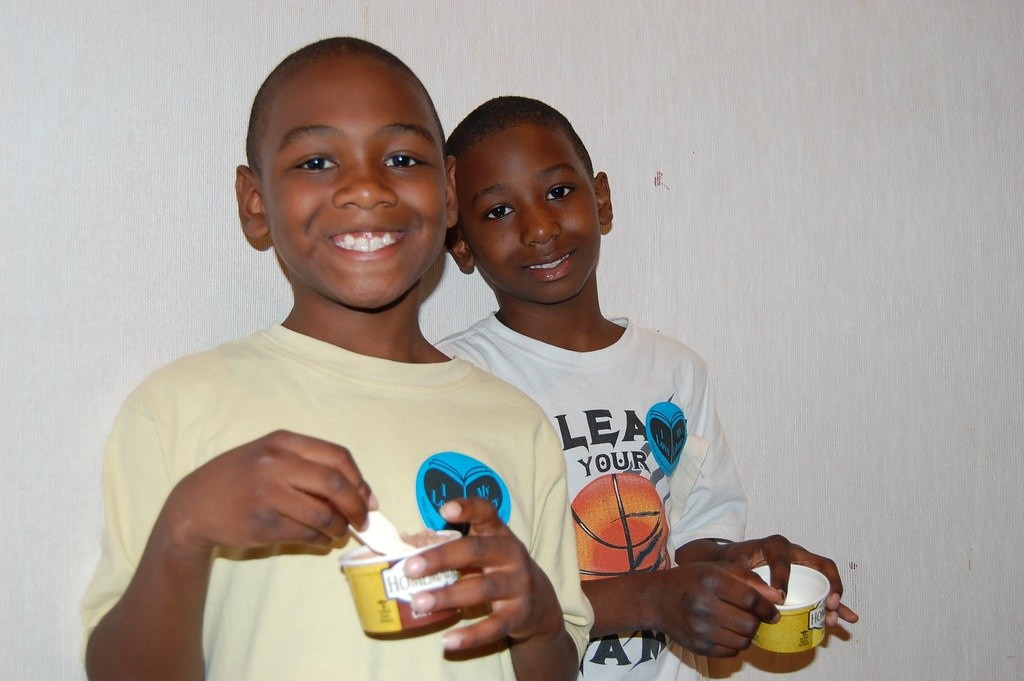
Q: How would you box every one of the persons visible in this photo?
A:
[443,95,859,681]
[80,36,596,681]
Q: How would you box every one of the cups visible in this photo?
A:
[751,564,830,653]
[337,529,461,633]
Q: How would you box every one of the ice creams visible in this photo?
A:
[337,527,465,634]
